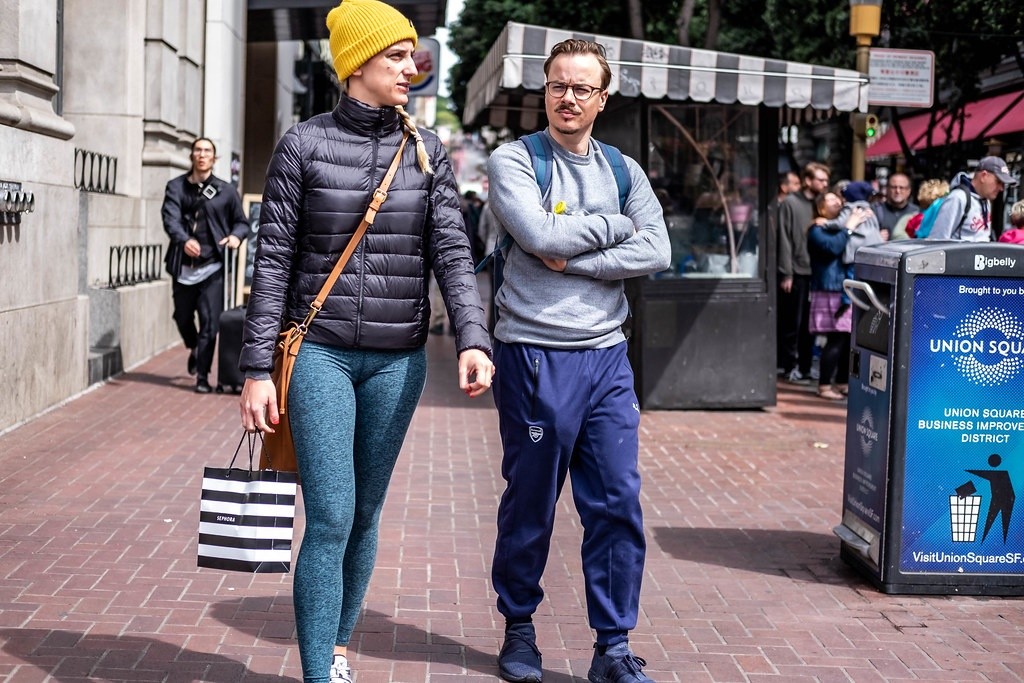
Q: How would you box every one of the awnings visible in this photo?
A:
[864,89,1024,155]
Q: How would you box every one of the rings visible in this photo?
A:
[490,380,493,383]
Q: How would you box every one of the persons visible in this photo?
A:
[237,0,495,683]
[161,138,250,391]
[488,39,672,683]
[457,190,498,276]
[701,158,1024,398]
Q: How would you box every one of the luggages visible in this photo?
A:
[215,242,250,395]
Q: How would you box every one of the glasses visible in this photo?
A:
[544,79,604,100]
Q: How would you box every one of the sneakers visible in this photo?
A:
[328,653,353,682]
[497,623,542,683]
[587,641,656,682]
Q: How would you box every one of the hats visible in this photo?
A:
[326,0,418,81]
[841,182,874,202]
[976,156,1017,187]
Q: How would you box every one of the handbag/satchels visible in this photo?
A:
[195,426,300,575]
[164,224,189,282]
[257,326,304,485]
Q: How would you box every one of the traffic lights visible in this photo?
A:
[853,113,878,138]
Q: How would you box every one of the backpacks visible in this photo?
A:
[915,185,971,239]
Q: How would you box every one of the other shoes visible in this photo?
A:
[188,348,199,375]
[817,386,845,400]
[786,368,810,384]
[196,373,212,393]
[836,384,848,396]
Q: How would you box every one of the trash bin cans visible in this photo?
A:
[832,238,1024,599]
